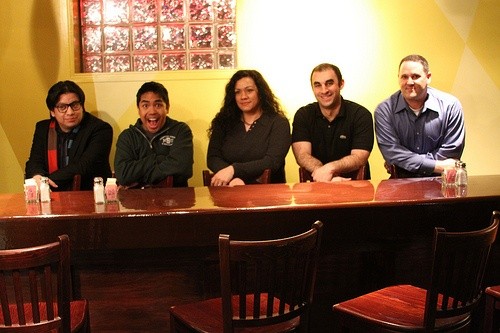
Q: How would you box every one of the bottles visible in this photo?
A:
[40,177,51,202]
[455,162,467,186]
[93,177,105,203]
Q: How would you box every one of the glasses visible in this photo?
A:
[54,100,83,113]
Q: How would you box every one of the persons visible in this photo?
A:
[374,54,466,178]
[25,80,114,192]
[206,69,291,187]
[114,81,194,189]
[290,63,374,183]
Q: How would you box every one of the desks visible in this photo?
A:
[1,174,500,298]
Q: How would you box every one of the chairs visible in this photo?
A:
[332,210,500,333]
[170,220,324,333]
[0,234,92,333]
